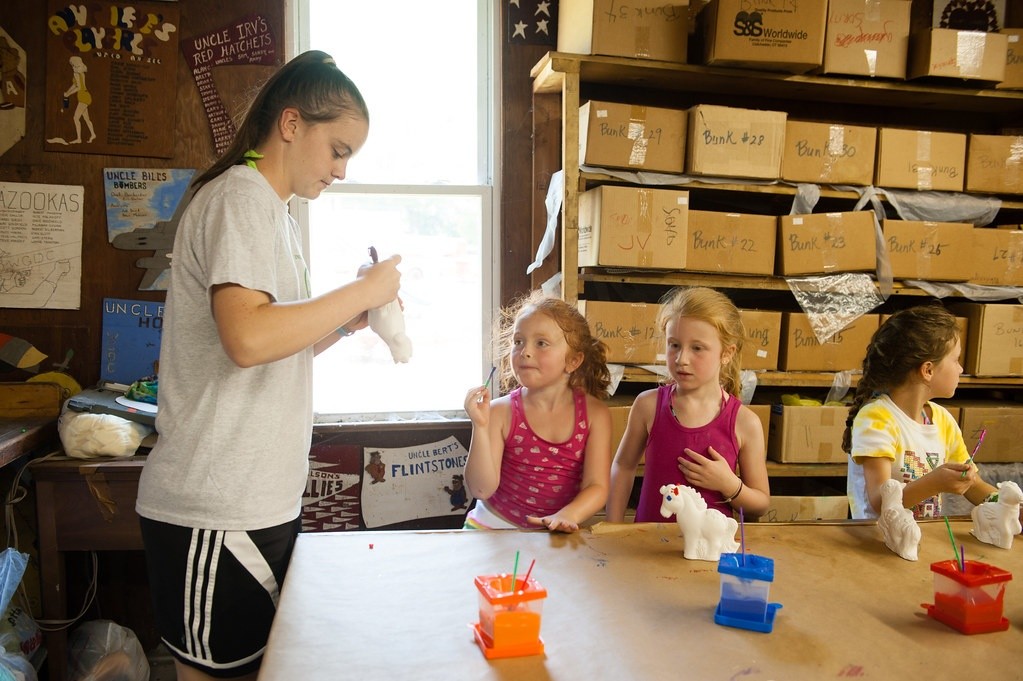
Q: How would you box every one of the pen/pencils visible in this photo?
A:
[367,247,379,264]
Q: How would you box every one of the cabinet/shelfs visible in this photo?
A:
[530,50,1023,529]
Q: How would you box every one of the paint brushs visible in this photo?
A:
[522,560,535,590]
[962,430,987,477]
[480,367,496,401]
[740,507,744,566]
[944,515,962,571]
[511,551,519,590]
[961,546,965,571]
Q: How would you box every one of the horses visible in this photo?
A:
[659,484,740,562]
[877,479,922,561]
[969,481,1023,550]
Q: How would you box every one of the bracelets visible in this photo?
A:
[724,479,743,503]
[983,490,998,502]
[336,327,355,337]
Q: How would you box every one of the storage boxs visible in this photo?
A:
[555,0,1023,527]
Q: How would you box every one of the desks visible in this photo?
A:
[0,382,158,681]
[260,515,1023,681]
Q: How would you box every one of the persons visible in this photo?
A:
[840,309,1023,535]
[606,288,771,523]
[462,298,611,533]
[135,50,403,681]
